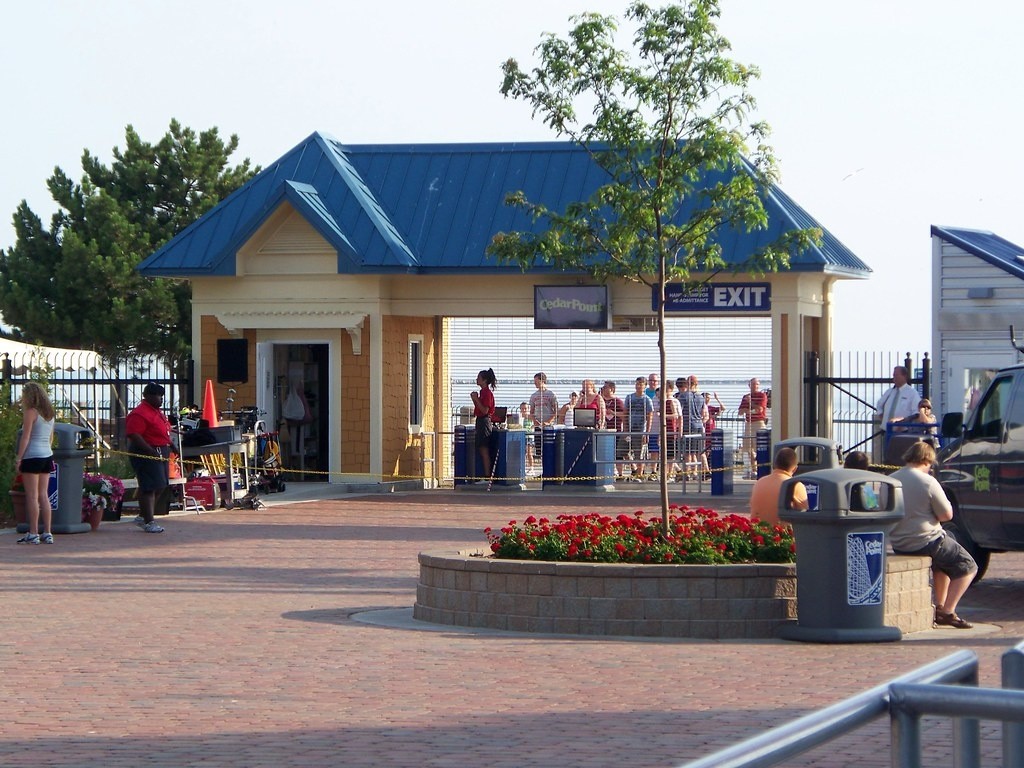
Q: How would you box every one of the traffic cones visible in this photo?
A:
[200,379,218,427]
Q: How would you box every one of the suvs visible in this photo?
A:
[931,363,1024,583]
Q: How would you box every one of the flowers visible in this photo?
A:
[10,473,26,492]
[94,473,125,512]
[81,473,116,523]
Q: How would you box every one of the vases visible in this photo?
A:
[8,490,26,523]
[101,498,123,522]
[89,509,104,532]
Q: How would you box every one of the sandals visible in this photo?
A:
[935,605,944,616]
[937,611,973,628]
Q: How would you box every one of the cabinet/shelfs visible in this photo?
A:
[287,360,320,457]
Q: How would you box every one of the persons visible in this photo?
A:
[844,451,880,510]
[520,402,534,472]
[908,399,940,451]
[738,378,767,479]
[877,365,921,435]
[14,382,56,545]
[879,442,978,629]
[750,447,809,526]
[529,372,557,455]
[470,368,496,485]
[125,382,171,533]
[559,374,725,482]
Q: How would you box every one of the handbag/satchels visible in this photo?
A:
[281,385,304,420]
[289,387,313,427]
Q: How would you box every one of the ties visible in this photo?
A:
[888,390,899,419]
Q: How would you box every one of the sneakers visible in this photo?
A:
[144,522,165,533]
[40,533,53,544]
[17,534,40,544]
[133,515,146,529]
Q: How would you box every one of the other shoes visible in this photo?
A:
[617,470,712,484]
[743,473,759,480]
[474,479,489,484]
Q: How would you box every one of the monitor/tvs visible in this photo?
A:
[490,406,508,426]
[574,408,596,427]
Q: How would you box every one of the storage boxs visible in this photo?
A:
[212,474,242,490]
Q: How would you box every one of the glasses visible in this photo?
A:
[921,405,931,410]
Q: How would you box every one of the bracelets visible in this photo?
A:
[16,460,21,464]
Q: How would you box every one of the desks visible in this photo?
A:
[182,442,249,504]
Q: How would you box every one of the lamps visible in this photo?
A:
[967,287,994,298]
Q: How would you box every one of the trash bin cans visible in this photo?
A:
[777,468,904,644]
[886,434,935,466]
[14,422,93,534]
[773,437,844,511]
